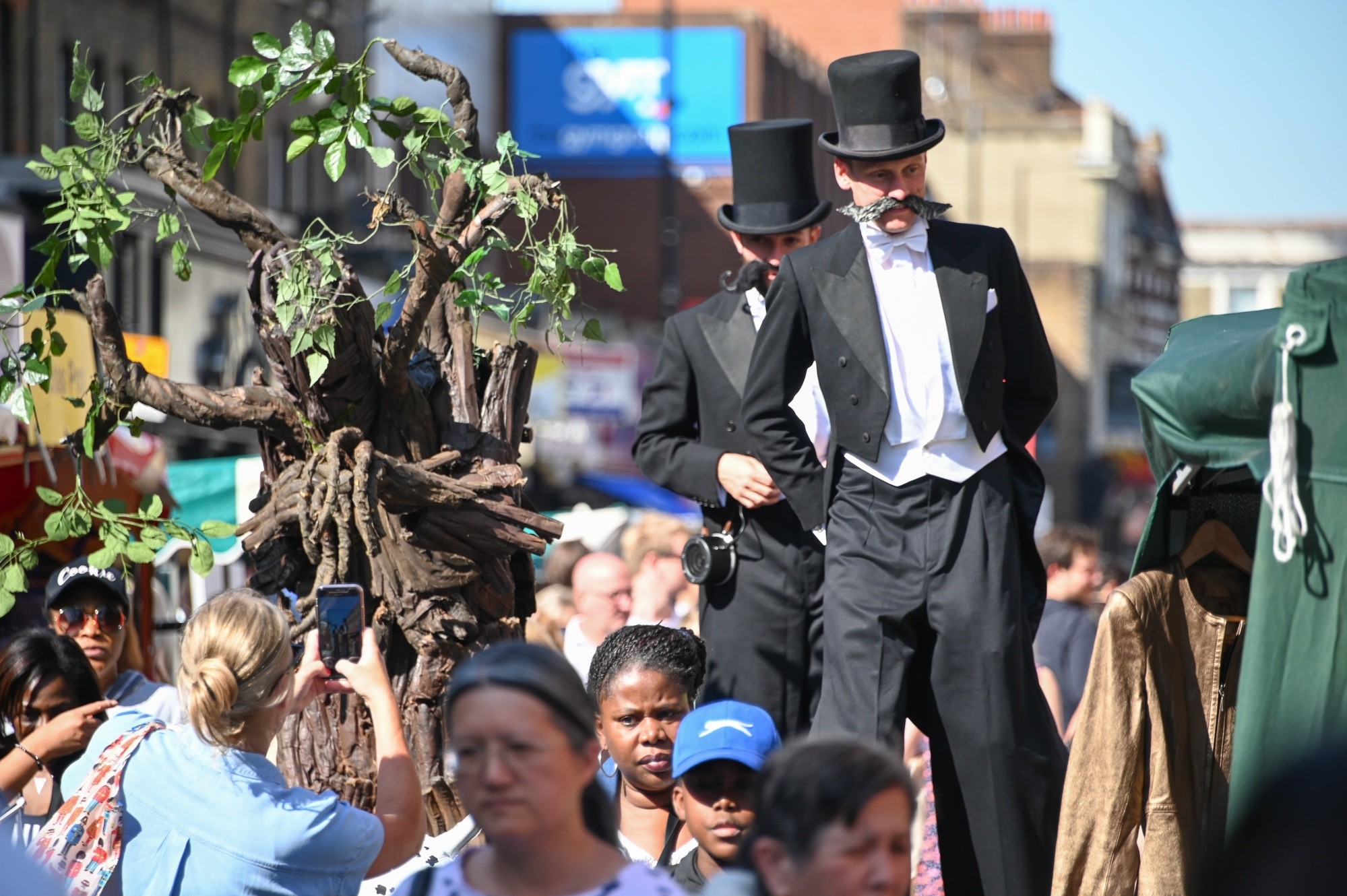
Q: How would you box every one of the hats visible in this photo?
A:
[717,117,834,236]
[671,700,784,779]
[817,50,946,163]
[44,556,130,621]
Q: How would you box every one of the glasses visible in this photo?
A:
[51,603,127,636]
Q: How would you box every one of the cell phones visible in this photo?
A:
[315,584,364,680]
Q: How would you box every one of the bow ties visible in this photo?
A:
[863,222,929,262]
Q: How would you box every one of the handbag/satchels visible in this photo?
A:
[22,720,169,896]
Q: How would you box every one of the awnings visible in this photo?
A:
[128,452,262,569]
[576,471,700,515]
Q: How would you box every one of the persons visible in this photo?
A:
[0,483,1148,896]
[740,45,1069,896]
[629,116,983,896]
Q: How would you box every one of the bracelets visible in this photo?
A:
[15,744,43,771]
